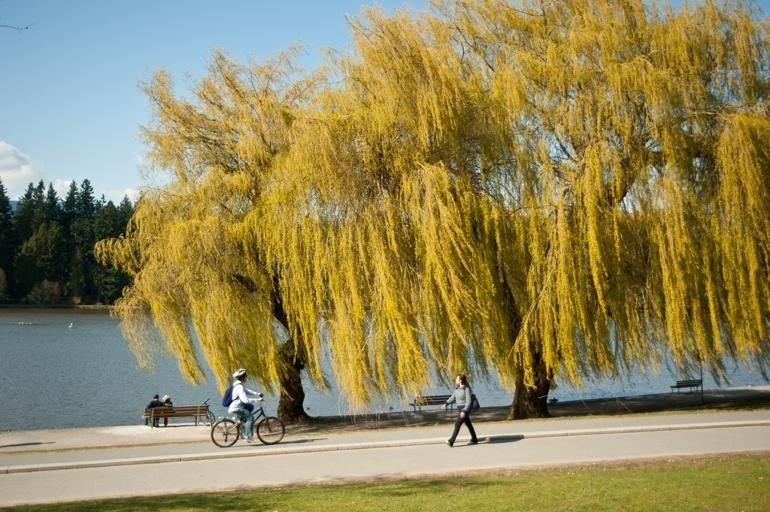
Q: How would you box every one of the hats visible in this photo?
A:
[162,395,171,401]
[153,394,159,399]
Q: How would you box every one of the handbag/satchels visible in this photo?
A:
[464,386,480,414]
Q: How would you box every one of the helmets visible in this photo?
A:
[232,368,246,378]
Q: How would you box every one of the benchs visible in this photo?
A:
[409,395,456,414]
[141,404,210,429]
[669,379,701,393]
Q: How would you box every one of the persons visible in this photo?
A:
[439,373,479,447]
[161,394,173,428]
[143,391,163,425]
[227,368,265,441]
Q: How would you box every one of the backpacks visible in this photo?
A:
[221,383,245,407]
[153,402,163,407]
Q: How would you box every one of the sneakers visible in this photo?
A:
[467,439,478,445]
[145,422,168,427]
[246,435,257,443]
[446,439,452,447]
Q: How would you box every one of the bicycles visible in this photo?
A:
[194,398,215,426]
[211,393,285,448]
